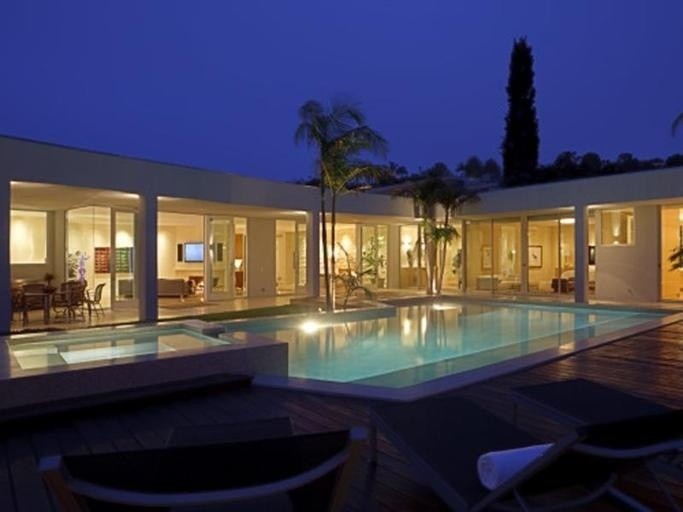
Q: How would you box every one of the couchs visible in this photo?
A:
[130,278,190,303]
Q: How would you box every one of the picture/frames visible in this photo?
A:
[528,245,543,269]
[480,243,493,269]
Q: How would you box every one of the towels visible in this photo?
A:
[477,442,556,490]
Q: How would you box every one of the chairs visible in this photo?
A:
[38,416,369,511]
[119,279,132,299]
[511,376,682,510]
[11,280,106,327]
[367,395,681,511]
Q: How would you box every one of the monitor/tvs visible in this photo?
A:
[184,242,204,262]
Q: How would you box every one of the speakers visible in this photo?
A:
[217,243,222,261]
[178,245,182,261]
